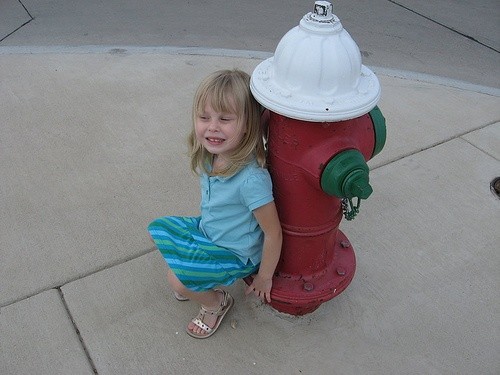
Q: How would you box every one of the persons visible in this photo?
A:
[149,67,284,339]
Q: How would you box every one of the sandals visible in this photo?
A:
[186,288,234,338]
[175,286,215,302]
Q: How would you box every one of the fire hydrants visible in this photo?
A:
[241,1,387,316]
